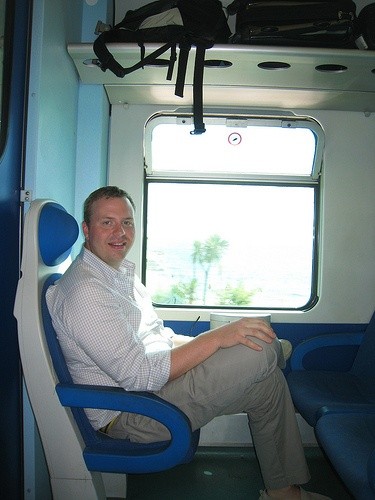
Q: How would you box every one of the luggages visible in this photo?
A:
[225,0,358,49]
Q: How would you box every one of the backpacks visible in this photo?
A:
[92,0,232,78]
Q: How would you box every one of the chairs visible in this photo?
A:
[286,311,375,500]
[12,199,200,500]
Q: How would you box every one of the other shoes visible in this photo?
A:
[277,338,293,361]
[259,487,331,500]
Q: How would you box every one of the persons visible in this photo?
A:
[43,185,334,500]
